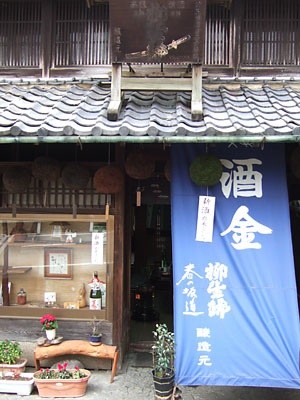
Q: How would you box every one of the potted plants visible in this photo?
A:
[0,339,91,397]
[151,324,176,400]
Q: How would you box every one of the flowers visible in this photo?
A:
[40,312,58,331]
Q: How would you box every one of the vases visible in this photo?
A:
[46,329,56,340]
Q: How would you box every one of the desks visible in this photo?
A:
[34,340,120,383]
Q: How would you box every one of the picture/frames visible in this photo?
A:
[44,248,73,279]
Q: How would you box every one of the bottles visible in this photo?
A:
[161,250,167,273]
[17,288,26,305]
[89,271,102,310]
